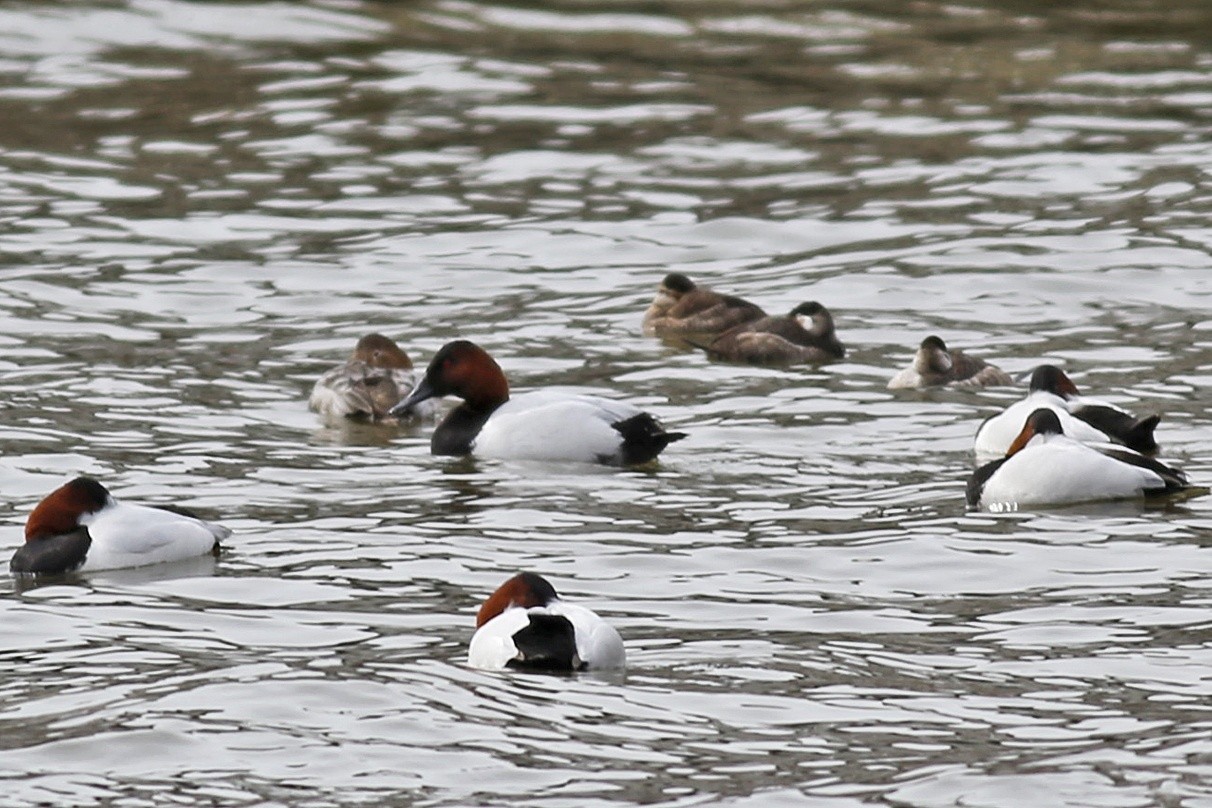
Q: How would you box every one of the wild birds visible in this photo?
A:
[640,273,846,369]
[965,365,1212,506]
[10,475,232,579]
[389,339,692,467]
[887,336,1031,394]
[467,572,626,674]
[308,334,465,427]
